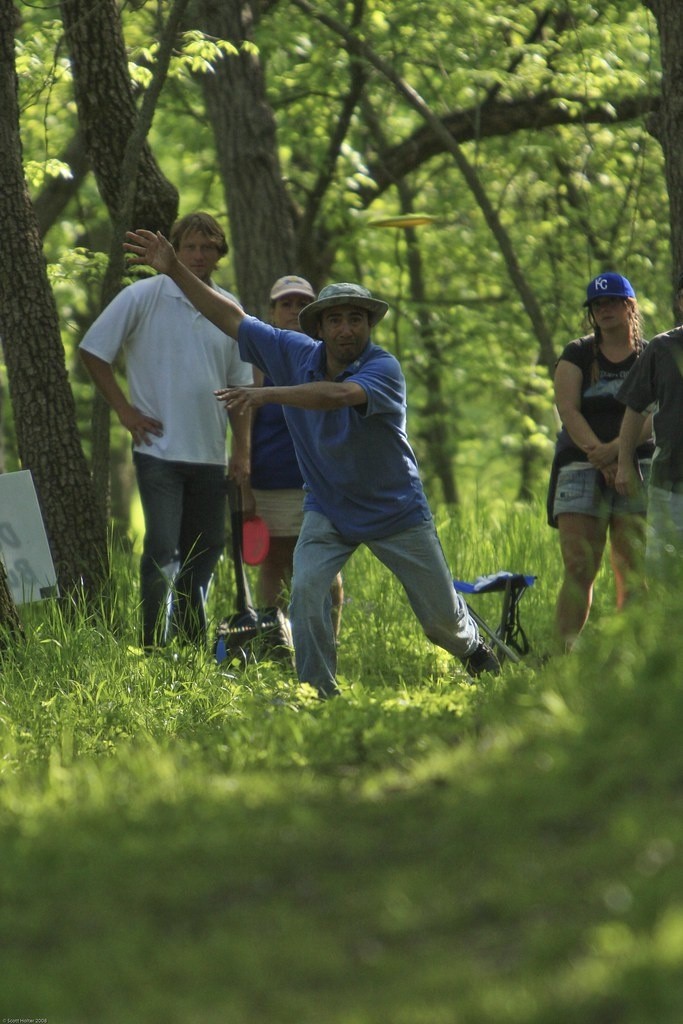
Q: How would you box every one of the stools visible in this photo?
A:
[451,570,536,666]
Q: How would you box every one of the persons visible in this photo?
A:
[74,215,260,653]
[118,222,502,697]
[540,275,654,658]
[222,267,345,637]
[613,284,683,596]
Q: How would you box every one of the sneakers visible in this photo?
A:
[459,636,501,681]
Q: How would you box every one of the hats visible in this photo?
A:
[297,283,389,341]
[268,275,316,304]
[582,272,636,308]
